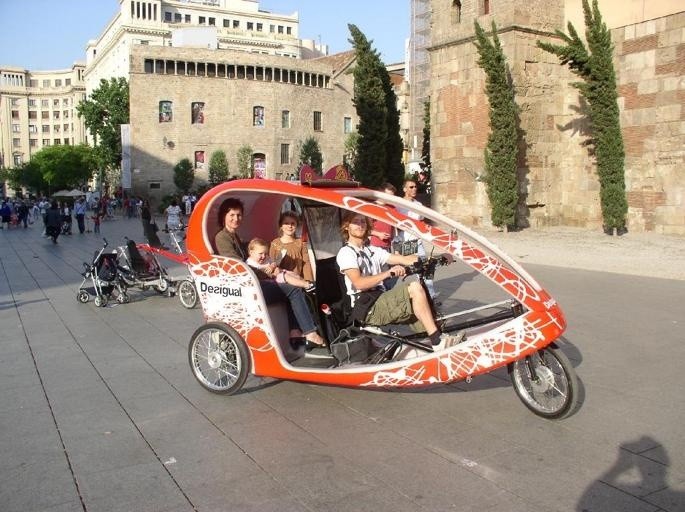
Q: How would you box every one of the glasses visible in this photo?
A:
[410,186,418,188]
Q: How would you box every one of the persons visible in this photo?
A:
[162,199,184,230]
[335,211,466,353]
[215,197,329,351]
[393,179,442,307]
[271,210,321,336]
[182,192,196,215]
[2,193,152,244]
[244,239,317,296]
[367,183,397,271]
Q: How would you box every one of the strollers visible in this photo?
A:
[78,235,176,306]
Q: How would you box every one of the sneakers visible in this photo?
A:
[305,280,319,292]
[432,331,465,352]
[433,298,441,306]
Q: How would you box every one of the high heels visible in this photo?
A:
[290,336,329,351]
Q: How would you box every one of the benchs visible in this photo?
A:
[266,301,315,362]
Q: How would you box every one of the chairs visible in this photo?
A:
[332,260,425,365]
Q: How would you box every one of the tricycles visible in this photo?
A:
[185,164,578,423]
